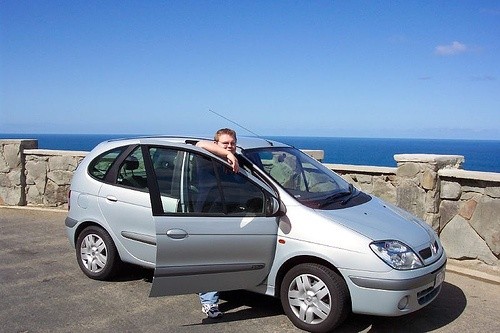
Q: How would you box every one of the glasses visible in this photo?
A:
[218,140,238,146]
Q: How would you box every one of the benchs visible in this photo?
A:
[122,151,174,193]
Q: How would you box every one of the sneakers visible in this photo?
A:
[201,304,223,319]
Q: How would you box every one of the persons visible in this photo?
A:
[195,129,240,319]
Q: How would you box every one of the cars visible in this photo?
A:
[64,135,447,333]
